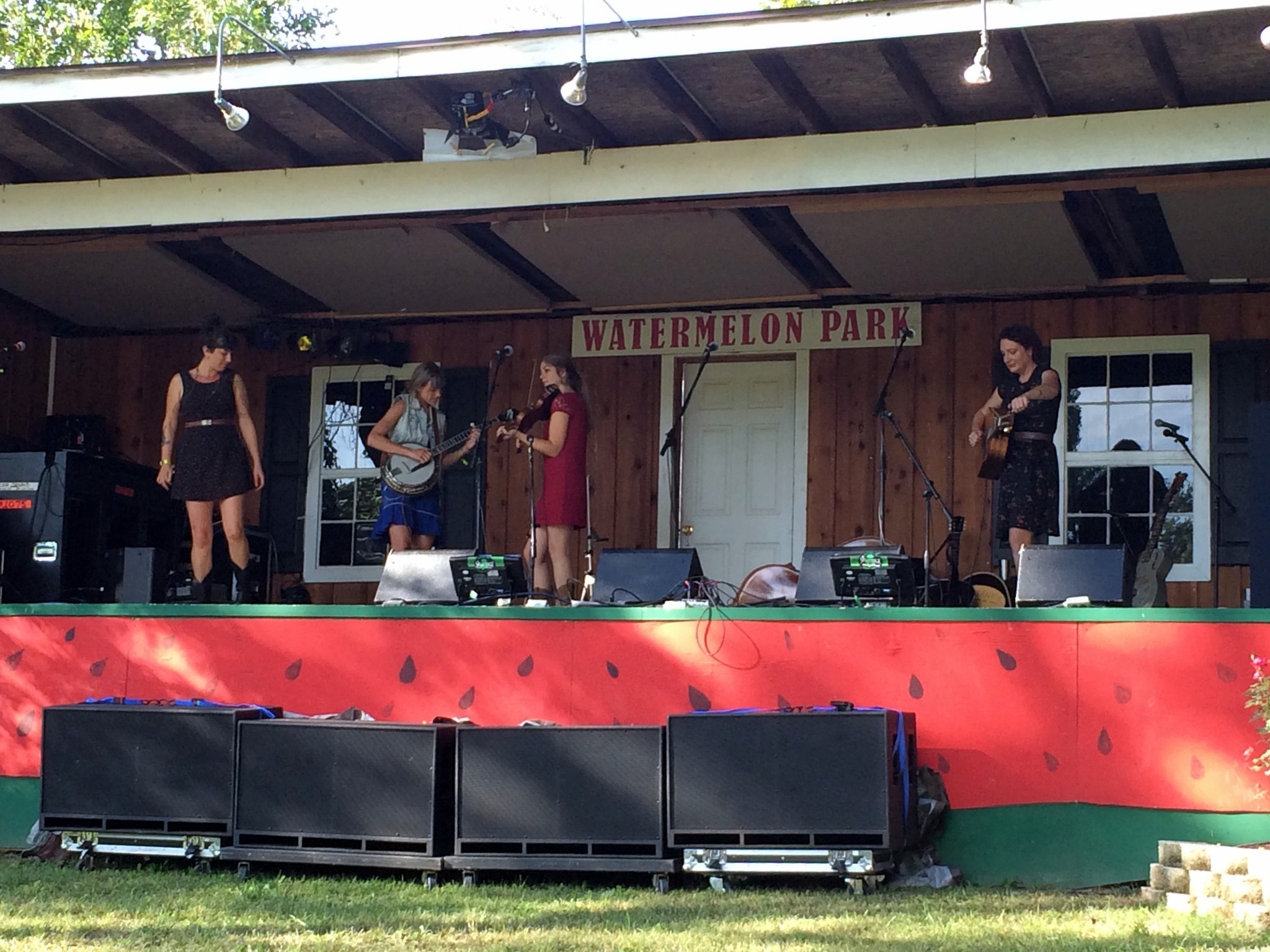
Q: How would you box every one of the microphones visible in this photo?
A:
[1101,509,1128,518]
[526,435,534,443]
[495,345,514,357]
[704,341,719,352]
[1155,418,1180,430]
[3,341,26,352]
[901,326,915,339]
[1163,429,1189,442]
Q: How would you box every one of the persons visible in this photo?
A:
[367,363,480,553]
[497,354,589,607]
[157,329,265,602]
[968,325,1061,575]
[1089,440,1169,558]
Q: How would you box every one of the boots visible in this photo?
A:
[228,557,255,604]
[192,570,212,603]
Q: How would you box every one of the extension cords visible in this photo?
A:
[1061,596,1090,608]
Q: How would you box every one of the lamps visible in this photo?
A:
[213,16,295,131]
[446,88,520,149]
[964,0,1012,84]
[286,328,315,353]
[560,0,639,105]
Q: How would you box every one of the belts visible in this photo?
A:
[184,419,233,427]
[1011,432,1053,442]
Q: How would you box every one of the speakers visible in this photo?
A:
[114,546,167,604]
[593,547,709,607]
[375,548,495,607]
[39,703,917,851]
[1016,544,1136,607]
[795,543,918,607]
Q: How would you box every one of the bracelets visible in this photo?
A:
[160,459,171,465]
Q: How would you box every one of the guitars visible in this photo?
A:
[1131,471,1189,607]
[972,406,1015,481]
[380,406,520,496]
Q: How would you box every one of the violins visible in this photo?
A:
[495,383,560,444]
